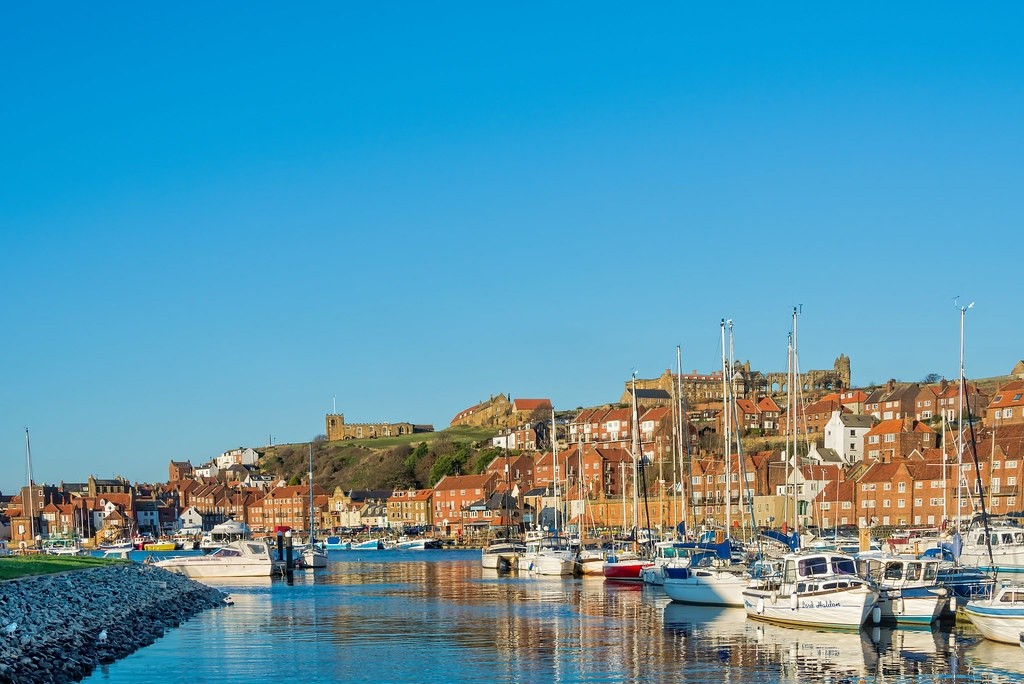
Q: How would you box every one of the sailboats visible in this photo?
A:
[481,295,1024,567]
[300,444,326,568]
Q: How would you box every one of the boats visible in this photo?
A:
[662,569,1024,645]
[0,531,195,559]
[143,539,272,588]
[322,536,445,550]
